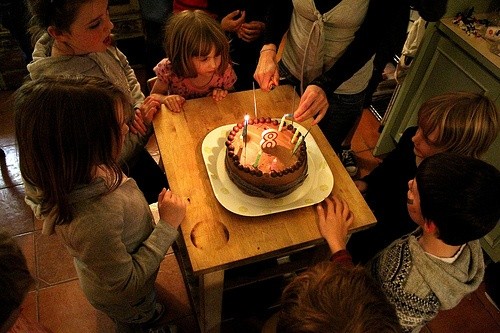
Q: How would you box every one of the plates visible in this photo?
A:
[202,119,333,216]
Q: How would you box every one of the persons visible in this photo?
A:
[253,0,449,177]
[364,153,500,333]
[263,196,401,333]
[345,91,500,264]
[14,76,185,325]
[208,0,294,92]
[27,0,170,205]
[149,10,237,113]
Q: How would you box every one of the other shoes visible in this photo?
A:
[143,303,164,325]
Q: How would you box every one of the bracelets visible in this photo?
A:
[261,48,276,52]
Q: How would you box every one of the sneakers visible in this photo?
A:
[338,146,358,176]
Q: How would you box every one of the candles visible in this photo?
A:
[242,113,305,168]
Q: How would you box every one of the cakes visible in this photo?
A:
[224,117,310,200]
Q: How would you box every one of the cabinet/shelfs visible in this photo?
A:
[372,11,500,263]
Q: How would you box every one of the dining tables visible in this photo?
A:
[151,84,378,333]
